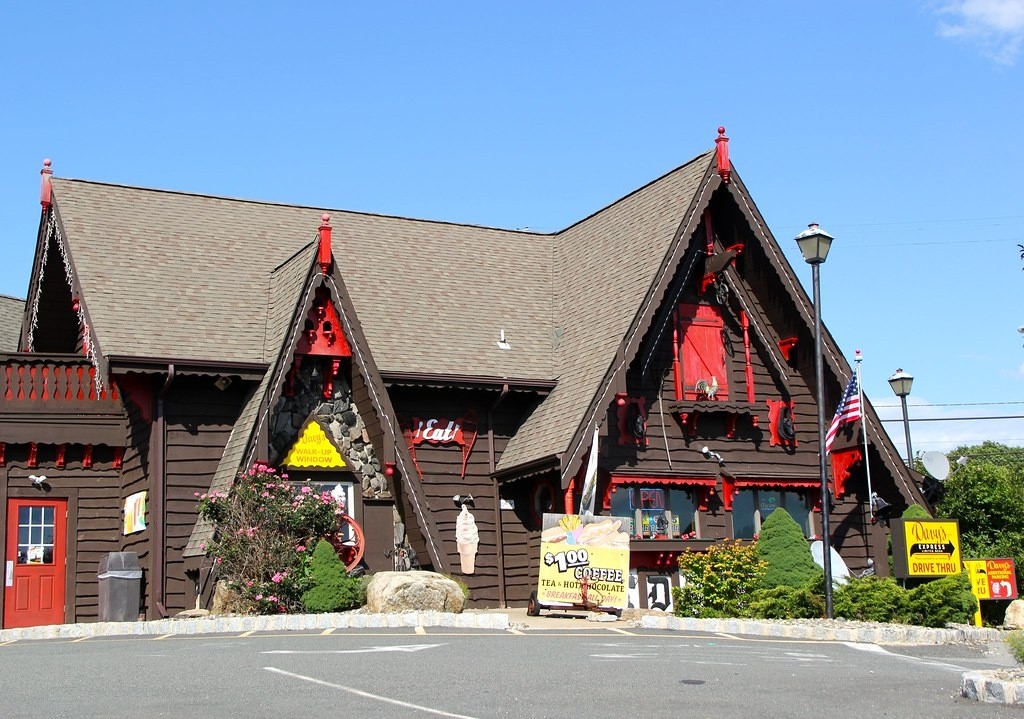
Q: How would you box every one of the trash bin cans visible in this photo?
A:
[96,552,143,621]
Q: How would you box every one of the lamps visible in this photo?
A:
[28,475,47,488]
[452,493,474,504]
[702,446,724,464]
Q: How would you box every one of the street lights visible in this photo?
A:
[795,221,836,621]
[886,366,914,470]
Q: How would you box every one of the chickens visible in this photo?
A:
[694,376,726,400]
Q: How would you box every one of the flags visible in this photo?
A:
[818,365,862,456]
